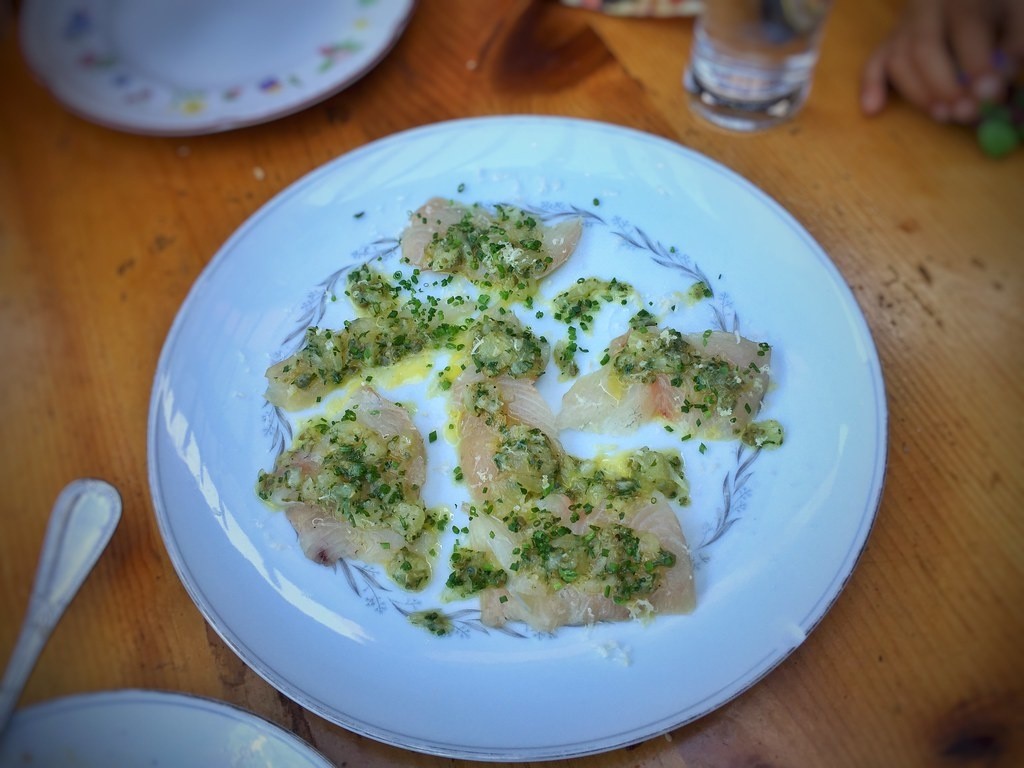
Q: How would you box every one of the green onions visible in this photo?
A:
[257,184,768,600]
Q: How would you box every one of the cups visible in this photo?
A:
[683,0,831,136]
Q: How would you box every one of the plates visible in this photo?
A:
[0,690,334,767]
[145,113,887,765]
[16,1,414,138]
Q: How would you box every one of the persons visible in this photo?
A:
[862,0,1024,118]
